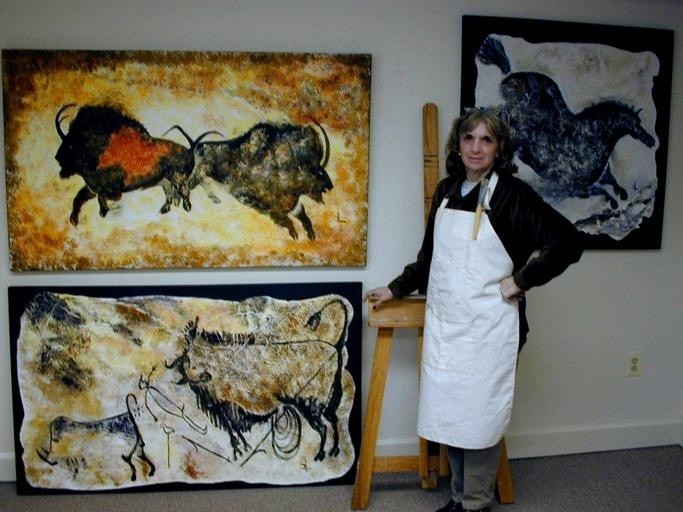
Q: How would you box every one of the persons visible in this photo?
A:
[362,109,583,512]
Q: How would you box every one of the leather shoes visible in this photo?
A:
[434,499,490,512]
